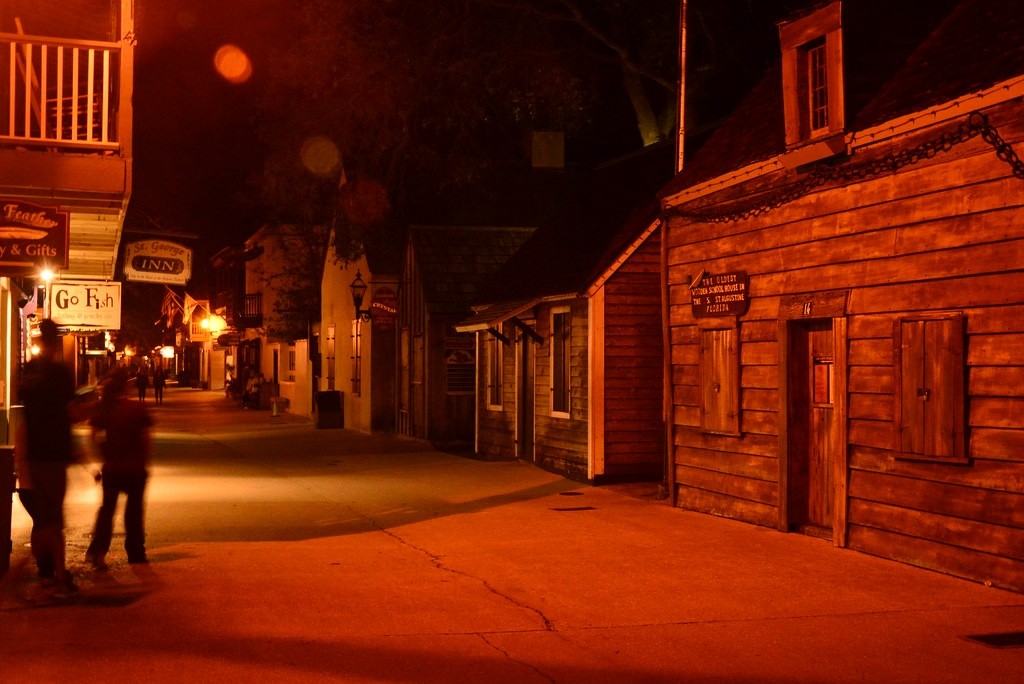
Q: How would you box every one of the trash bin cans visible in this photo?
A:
[313,389,343,430]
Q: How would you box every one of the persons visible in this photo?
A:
[84,364,154,572]
[243,372,266,411]
[12,318,94,598]
[118,361,168,405]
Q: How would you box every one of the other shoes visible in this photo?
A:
[40,576,79,598]
[84,552,109,570]
[127,550,149,564]
